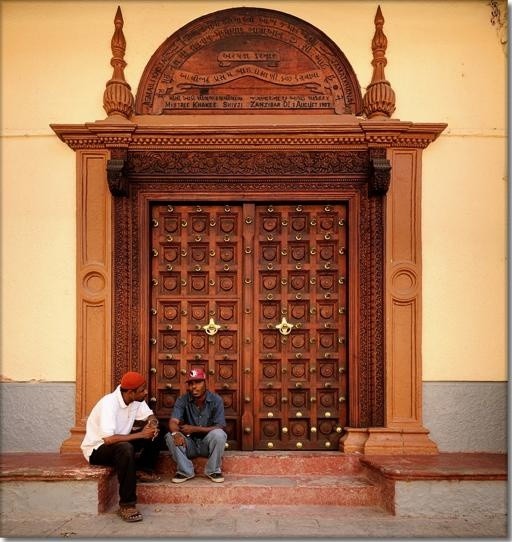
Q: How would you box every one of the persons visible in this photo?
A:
[161,367,228,484]
[77,371,165,523]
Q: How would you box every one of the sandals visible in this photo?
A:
[134,468,163,482]
[119,504,144,524]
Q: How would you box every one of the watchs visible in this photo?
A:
[171,432,180,436]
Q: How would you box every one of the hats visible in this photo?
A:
[120,370,146,391]
[183,367,208,384]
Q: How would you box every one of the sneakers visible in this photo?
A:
[207,472,225,484]
[171,471,196,484]
